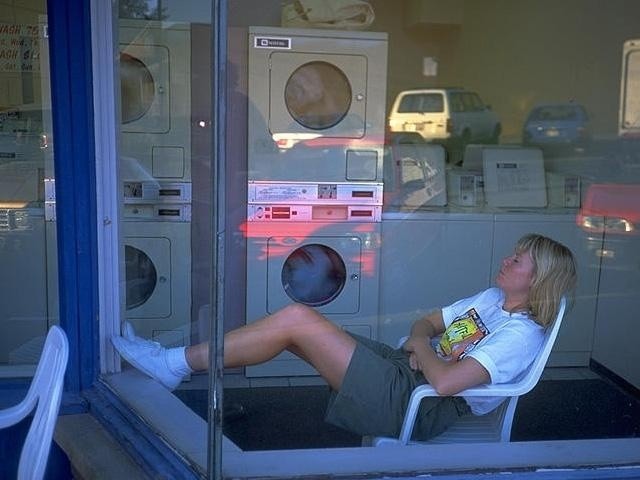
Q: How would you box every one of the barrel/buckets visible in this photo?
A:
[0,204,46,379]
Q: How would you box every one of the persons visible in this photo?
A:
[110,232,577,441]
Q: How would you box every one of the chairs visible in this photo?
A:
[0,326,70,480]
[363,289,567,446]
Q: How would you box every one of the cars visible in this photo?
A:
[1,99,59,236]
[523,101,640,290]
[232,113,448,281]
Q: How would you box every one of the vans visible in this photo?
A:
[385,86,502,145]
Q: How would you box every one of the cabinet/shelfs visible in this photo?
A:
[382,207,606,368]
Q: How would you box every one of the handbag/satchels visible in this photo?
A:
[281,0,375,30]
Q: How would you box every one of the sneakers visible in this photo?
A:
[110,320,182,393]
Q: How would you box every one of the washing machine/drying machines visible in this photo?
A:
[245,206,380,378]
[121,205,192,350]
[380,141,496,372]
[491,144,604,369]
[118,18,190,205]
[246,24,388,204]
